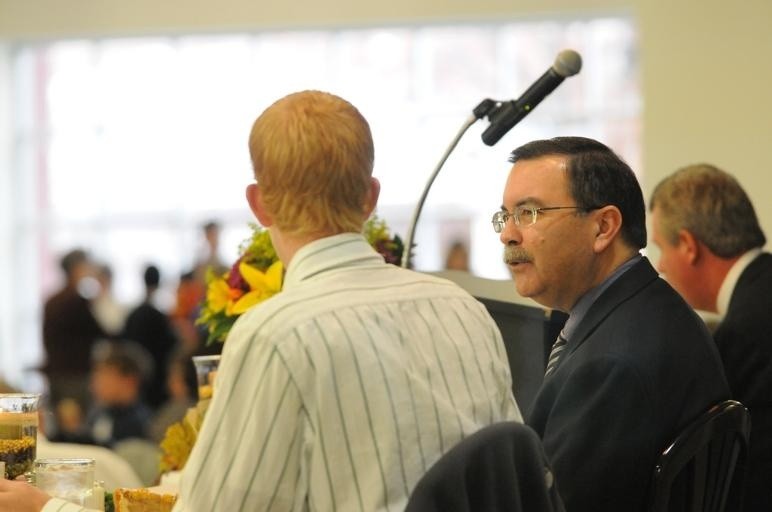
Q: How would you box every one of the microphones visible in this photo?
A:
[481,49,582,146]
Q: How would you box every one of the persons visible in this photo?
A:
[646,162,770,509]
[1,89,528,511]
[36,215,238,450]
[440,239,475,274]
[491,135,735,509]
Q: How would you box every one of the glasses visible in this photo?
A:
[492,205,593,233]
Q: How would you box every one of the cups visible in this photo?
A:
[33,457,95,512]
[0,393,40,484]
[193,354,224,407]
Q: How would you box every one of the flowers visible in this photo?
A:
[196,202,415,347]
